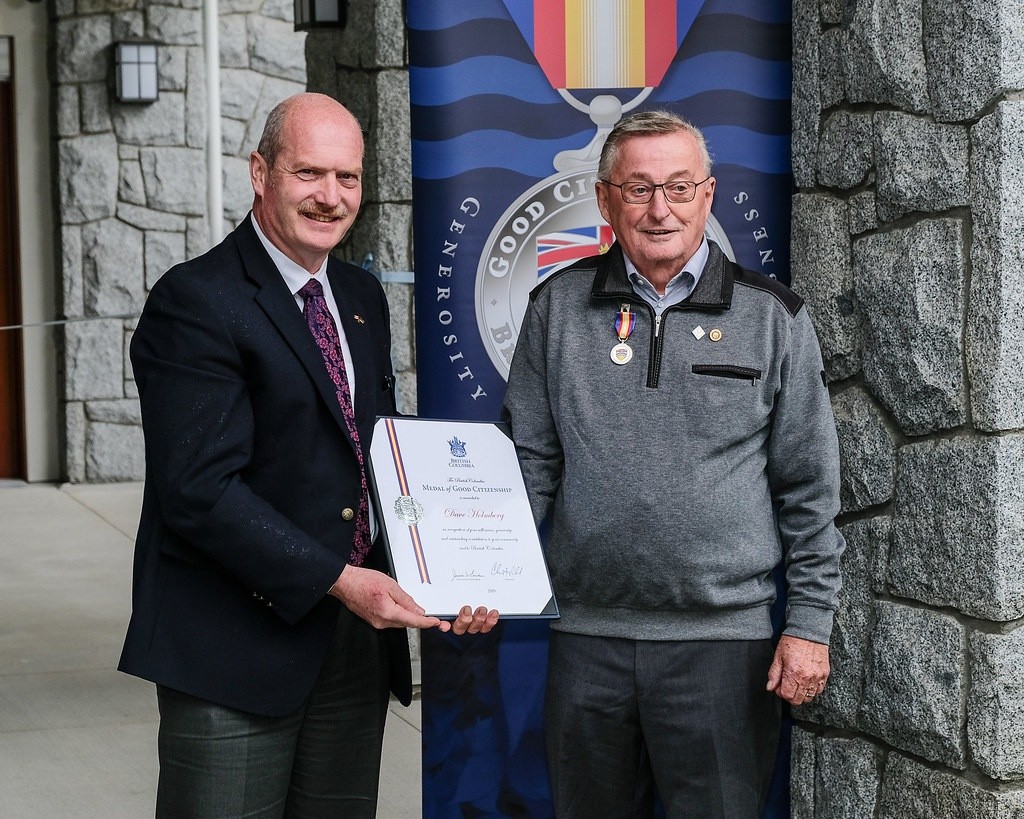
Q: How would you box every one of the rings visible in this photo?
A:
[806,693,815,699]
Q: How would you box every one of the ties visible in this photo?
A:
[297,278,372,567]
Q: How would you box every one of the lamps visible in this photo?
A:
[109,38,159,104]
[293,0,349,31]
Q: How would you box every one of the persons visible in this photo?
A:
[440,112,846,819]
[116,93,440,819]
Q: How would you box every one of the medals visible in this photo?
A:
[609,343,632,365]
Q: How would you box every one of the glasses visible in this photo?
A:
[600,177,709,204]
